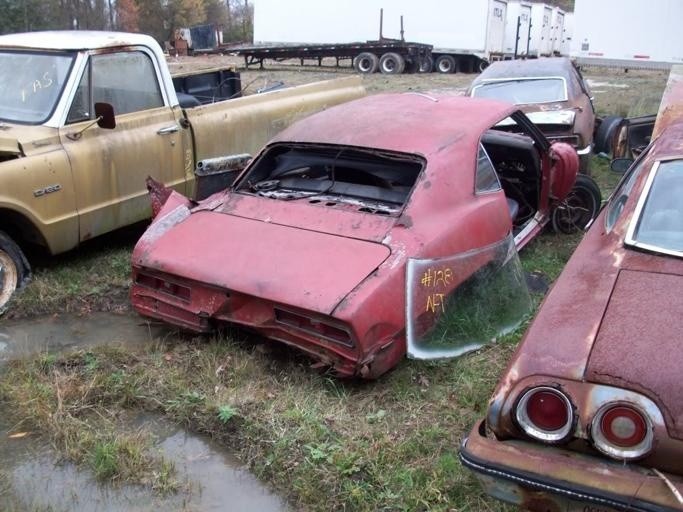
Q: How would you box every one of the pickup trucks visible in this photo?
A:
[0,30,363,318]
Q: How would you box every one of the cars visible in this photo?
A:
[460,61,683,512]
[132,88,601,385]
[464,60,596,176]
